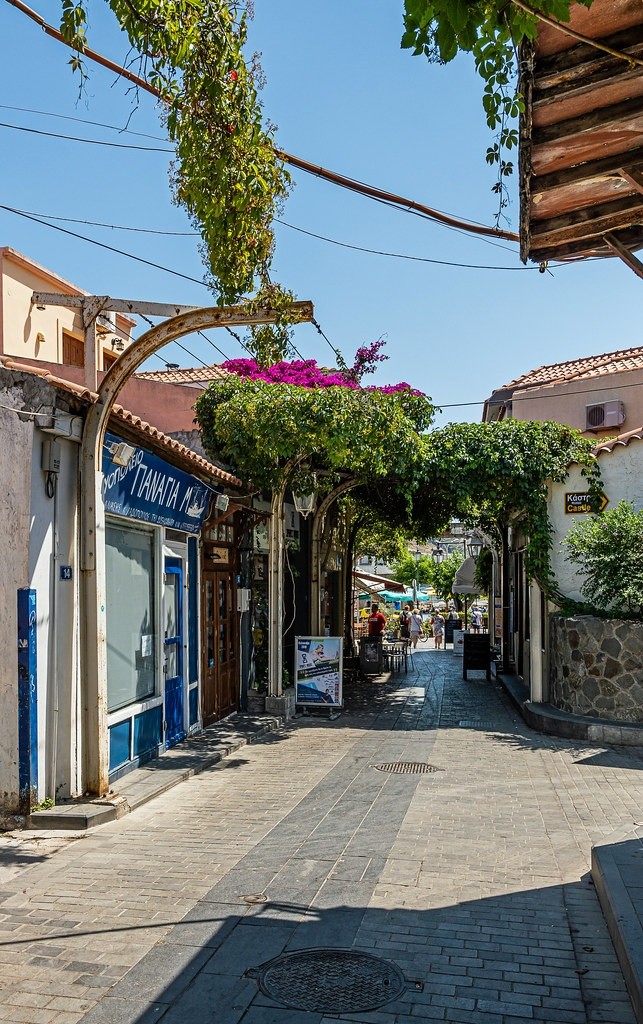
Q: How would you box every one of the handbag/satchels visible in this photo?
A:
[419,626,423,635]
[471,612,477,623]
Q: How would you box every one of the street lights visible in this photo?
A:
[432,539,468,630]
[415,549,421,610]
[290,470,321,636]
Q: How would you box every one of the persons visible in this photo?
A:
[398,605,459,649]
[310,645,334,662]
[368,604,386,636]
[471,608,482,634]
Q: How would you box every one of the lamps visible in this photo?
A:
[215,494,230,512]
[111,337,124,351]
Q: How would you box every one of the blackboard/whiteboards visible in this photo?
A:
[444,619,463,643]
[463,632,492,670]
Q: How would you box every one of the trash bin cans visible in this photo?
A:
[358,635,384,676]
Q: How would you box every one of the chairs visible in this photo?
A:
[353,623,413,676]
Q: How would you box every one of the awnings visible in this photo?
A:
[351,569,404,602]
[452,557,486,594]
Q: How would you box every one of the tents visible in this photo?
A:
[359,585,428,602]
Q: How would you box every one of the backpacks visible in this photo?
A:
[401,611,410,625]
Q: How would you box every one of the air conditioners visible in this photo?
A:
[585,400,626,434]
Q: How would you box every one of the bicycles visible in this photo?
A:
[418,629,429,642]
